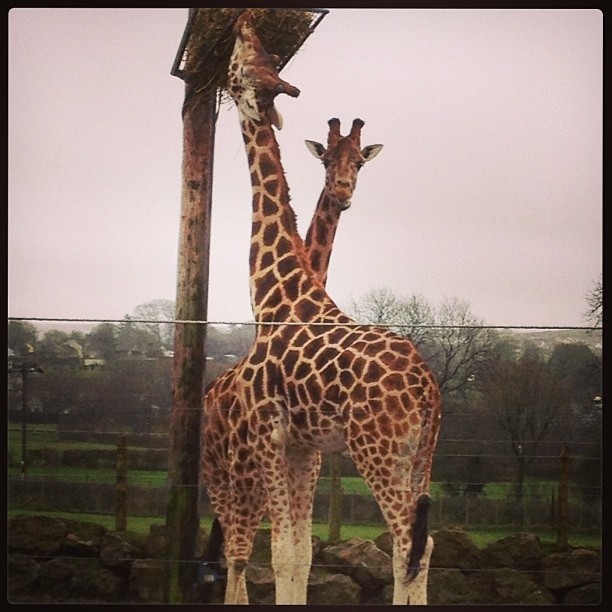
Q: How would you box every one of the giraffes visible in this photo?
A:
[224,11,443,609]
[197,116,386,567]
